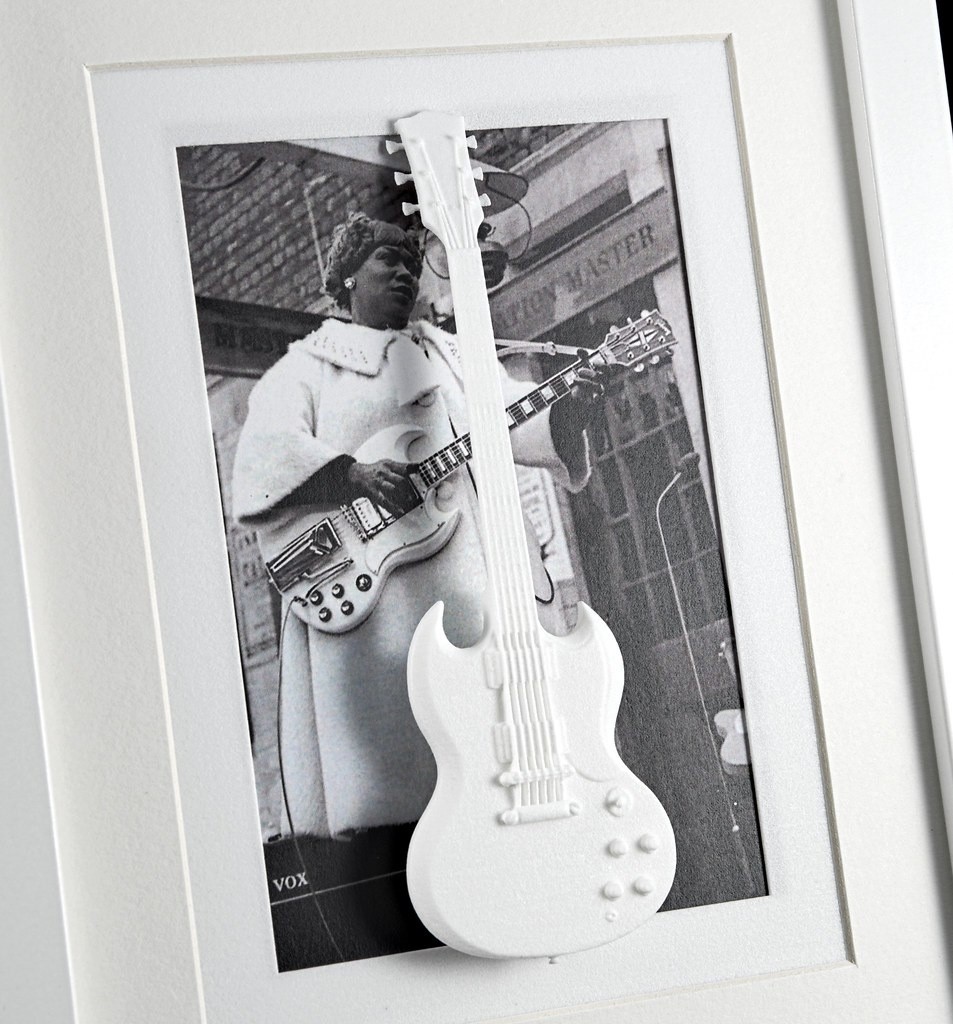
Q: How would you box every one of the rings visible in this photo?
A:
[599,384,606,398]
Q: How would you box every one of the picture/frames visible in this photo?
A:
[0,0,953,1024]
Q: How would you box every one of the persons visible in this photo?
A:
[234,210,609,953]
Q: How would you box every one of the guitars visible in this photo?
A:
[389,100,685,967]
[254,307,680,637]
